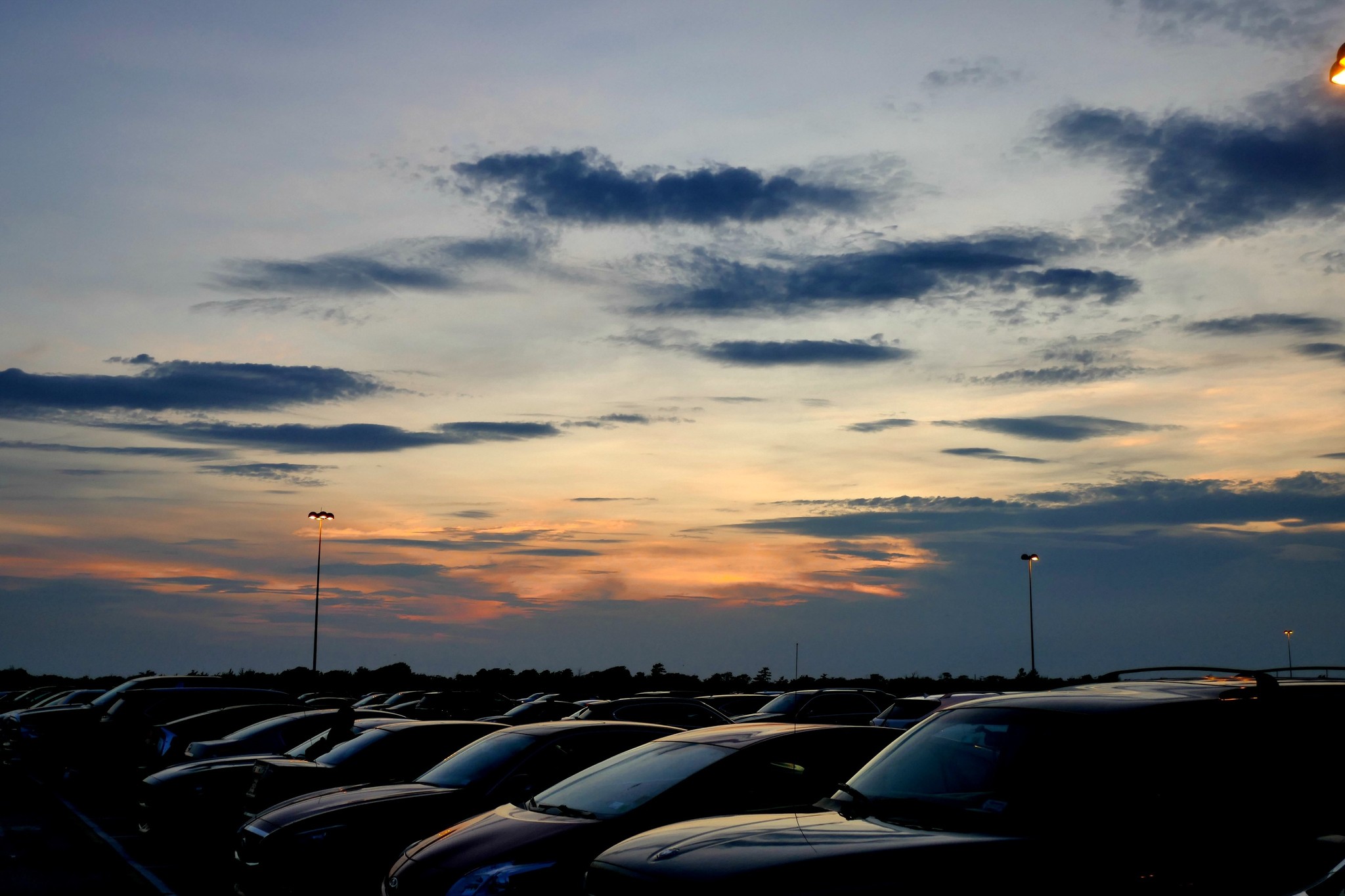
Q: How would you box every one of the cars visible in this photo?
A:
[0,666,1345,896]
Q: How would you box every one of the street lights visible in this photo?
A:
[1021,553,1039,677]
[1284,630,1294,677]
[307,511,335,670]
